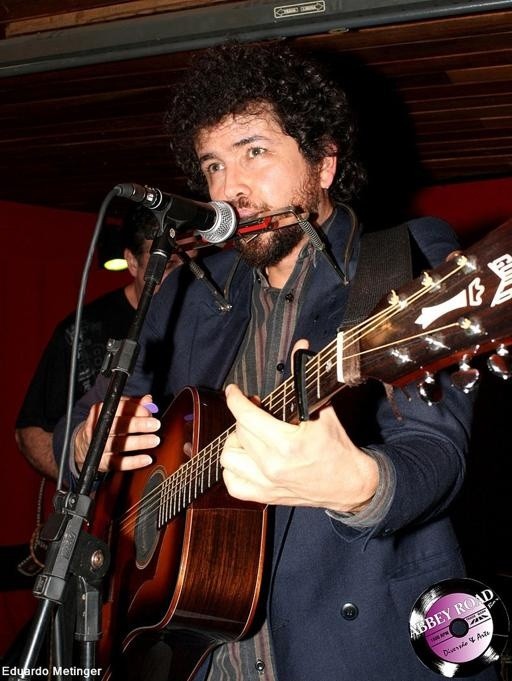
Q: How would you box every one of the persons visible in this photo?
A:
[15,202,200,496]
[52,31,501,681]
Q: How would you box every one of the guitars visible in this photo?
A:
[76,222,512,678]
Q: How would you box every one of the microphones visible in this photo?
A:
[117,182,239,244]
